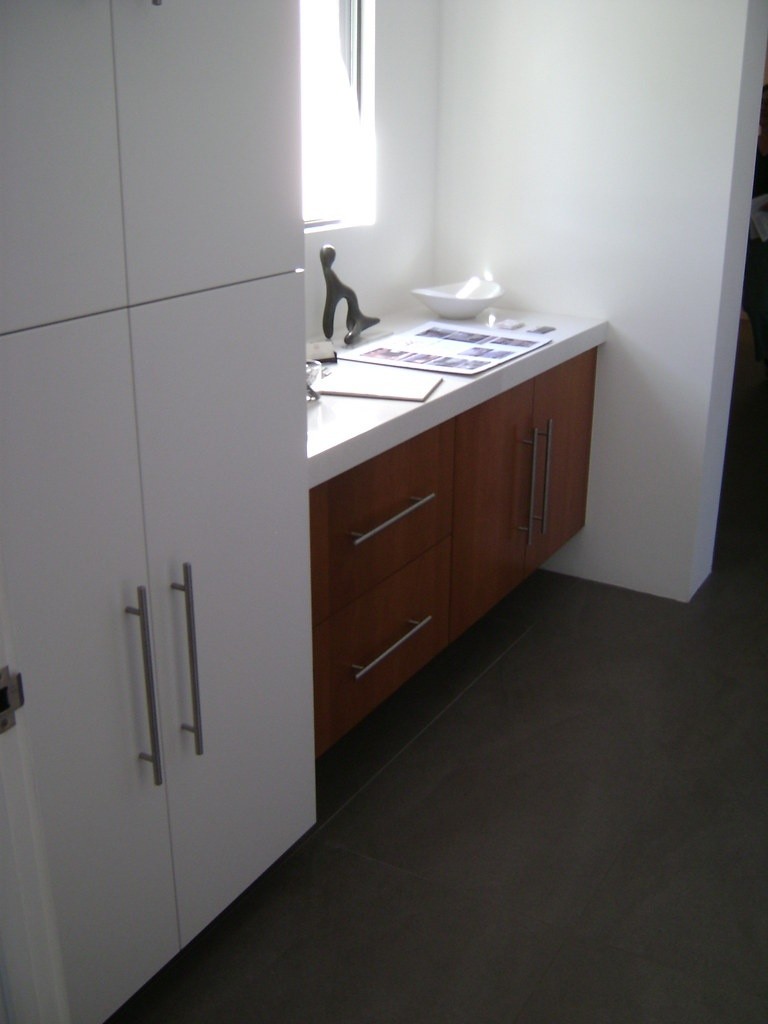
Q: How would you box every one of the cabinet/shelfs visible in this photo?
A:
[0,0,305,335]
[309,345,598,761]
[0,267,317,1024]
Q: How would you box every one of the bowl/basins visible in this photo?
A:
[411,281,501,317]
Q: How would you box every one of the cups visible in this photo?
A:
[305,360,323,403]
